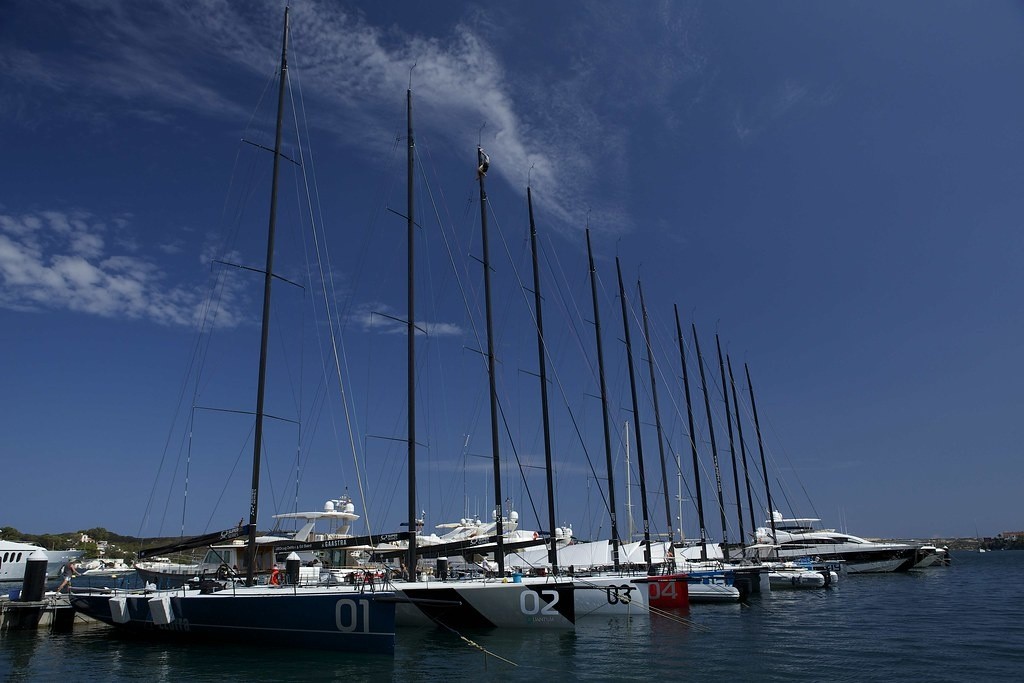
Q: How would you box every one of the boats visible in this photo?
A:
[0,538,88,581]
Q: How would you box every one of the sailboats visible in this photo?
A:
[69,0,464,646]
[128,58,946,629]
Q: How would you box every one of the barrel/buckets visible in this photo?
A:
[512,573,523,583]
[9,589,21,600]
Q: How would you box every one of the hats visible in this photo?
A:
[69,557,77,563]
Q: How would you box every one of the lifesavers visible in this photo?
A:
[270,570,285,585]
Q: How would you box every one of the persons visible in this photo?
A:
[56,556,81,593]
[307,559,318,567]
[272,564,278,572]
